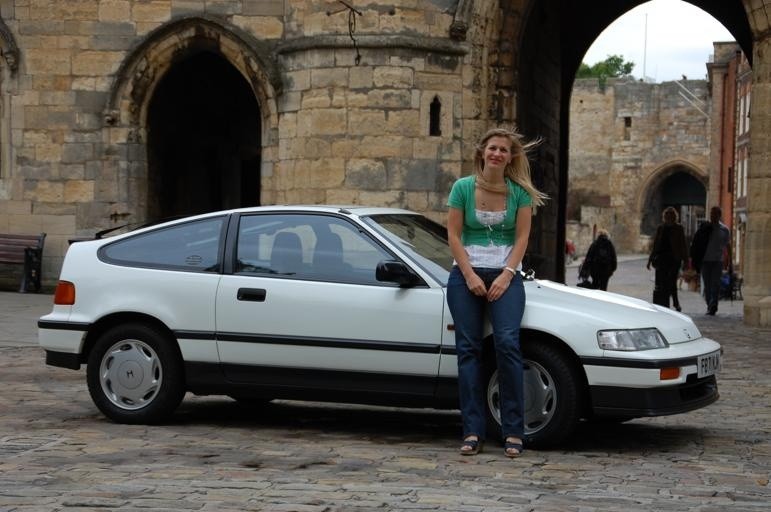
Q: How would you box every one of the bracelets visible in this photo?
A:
[505,267,516,276]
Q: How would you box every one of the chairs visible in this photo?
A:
[312,233,354,278]
[270,231,304,273]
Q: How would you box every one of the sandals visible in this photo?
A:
[459,438,481,455]
[504,438,524,457]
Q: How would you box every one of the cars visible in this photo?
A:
[38,205,723,450]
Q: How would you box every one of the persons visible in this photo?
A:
[447,128,552,458]
[646,207,732,315]
[576,229,617,291]
